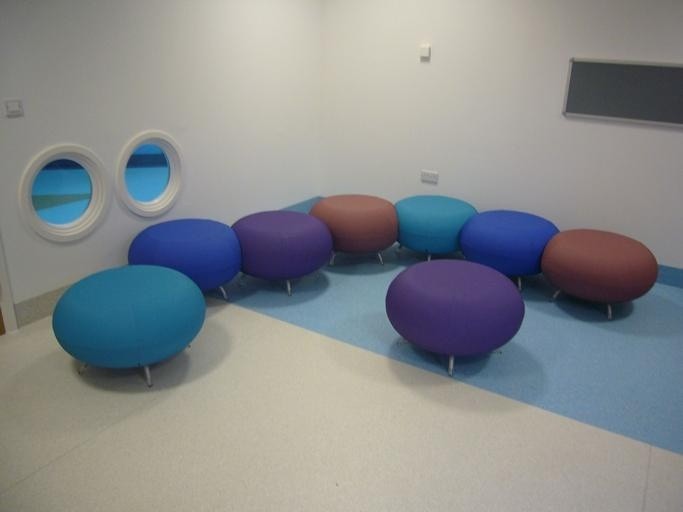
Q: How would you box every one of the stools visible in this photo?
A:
[231,211,334,295]
[459,210,559,291]
[53,265,206,387]
[386,259,524,376]
[309,194,398,266]
[395,196,477,261]
[541,229,658,320]
[128,218,241,300]
